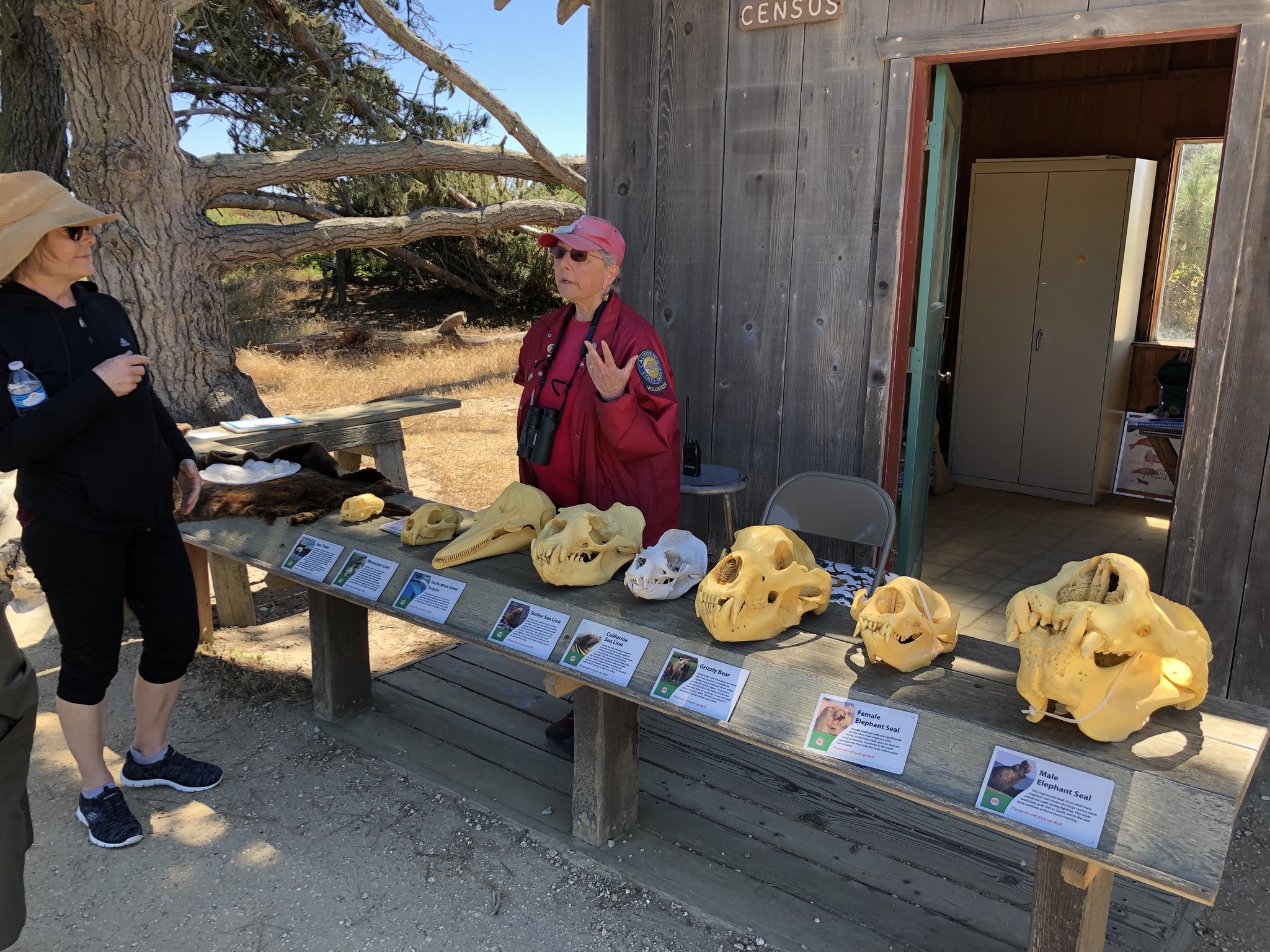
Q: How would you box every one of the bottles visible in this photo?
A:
[7,360,50,416]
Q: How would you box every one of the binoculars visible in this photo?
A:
[515,405,561,467]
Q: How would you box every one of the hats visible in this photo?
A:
[0,170,117,281]
[538,215,626,268]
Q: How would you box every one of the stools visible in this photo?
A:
[675,463,749,554]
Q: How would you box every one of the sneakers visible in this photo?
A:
[76,787,143,847]
[545,708,574,745]
[121,744,223,793]
[563,742,574,759]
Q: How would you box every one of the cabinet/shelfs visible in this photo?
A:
[946,155,1156,506]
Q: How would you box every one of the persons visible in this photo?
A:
[513,214,683,549]
[0,169,225,849]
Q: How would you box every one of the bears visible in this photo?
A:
[665,658,690,682]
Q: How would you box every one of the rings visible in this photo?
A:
[590,364,595,369]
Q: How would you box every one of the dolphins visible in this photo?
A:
[410,578,429,599]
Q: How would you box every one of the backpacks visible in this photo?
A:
[1156,352,1192,419]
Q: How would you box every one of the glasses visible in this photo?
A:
[67,225,89,240]
[550,245,605,263]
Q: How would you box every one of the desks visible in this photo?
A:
[181,484,1269,952]
[176,395,461,627]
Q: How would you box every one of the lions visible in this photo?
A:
[342,553,368,577]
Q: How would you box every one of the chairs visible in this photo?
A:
[759,472,897,634]
